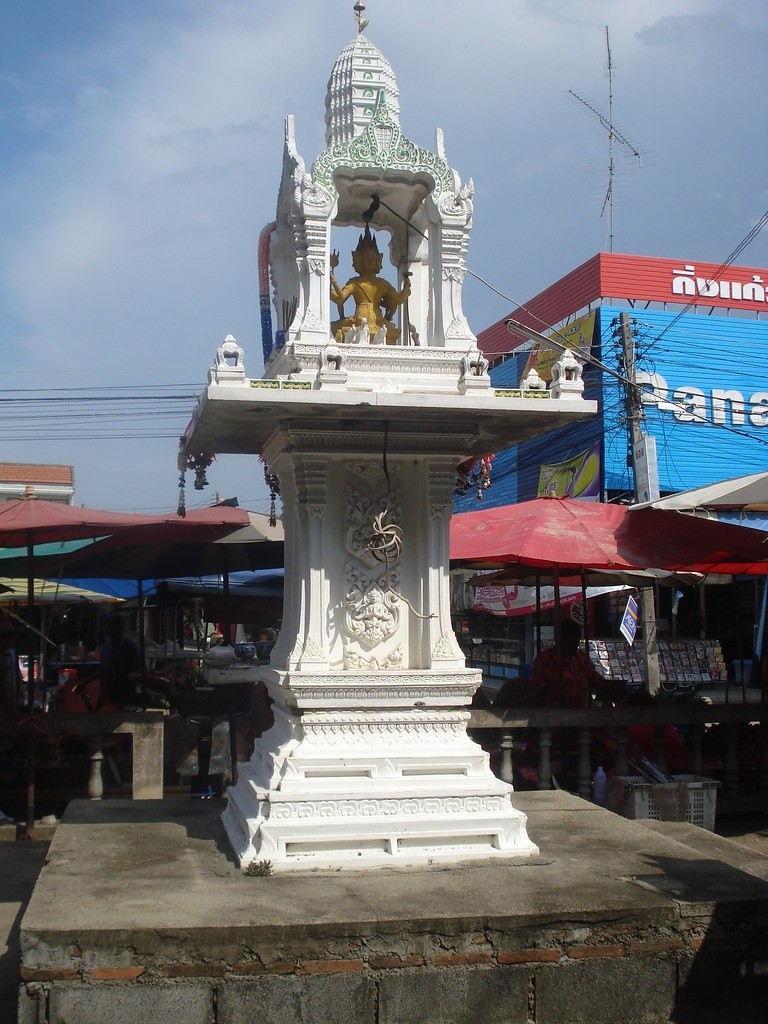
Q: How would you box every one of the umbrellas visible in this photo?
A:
[0,470,767,718]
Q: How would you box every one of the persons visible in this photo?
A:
[525,618,607,746]
[100,616,145,707]
[330,220,411,345]
[0,628,24,721]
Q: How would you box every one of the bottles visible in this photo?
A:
[593,767,607,804]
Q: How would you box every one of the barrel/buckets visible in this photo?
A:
[733,660,752,686]
